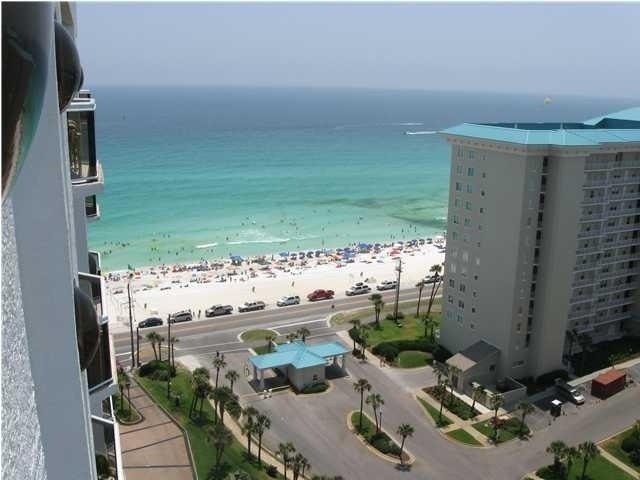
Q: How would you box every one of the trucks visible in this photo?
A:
[590,368,632,399]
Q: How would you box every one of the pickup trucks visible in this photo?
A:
[239,301,265,312]
[205,304,233,316]
[308,289,333,300]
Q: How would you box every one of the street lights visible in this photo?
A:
[125,273,135,368]
[126,383,132,420]
[166,376,171,397]
[392,256,402,321]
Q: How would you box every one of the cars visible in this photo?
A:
[421,275,440,282]
[139,318,163,327]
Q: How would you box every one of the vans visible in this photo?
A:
[276,295,301,306]
[555,382,585,405]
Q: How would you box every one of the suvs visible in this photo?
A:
[170,310,192,323]
[378,279,397,290]
[345,283,369,296]
